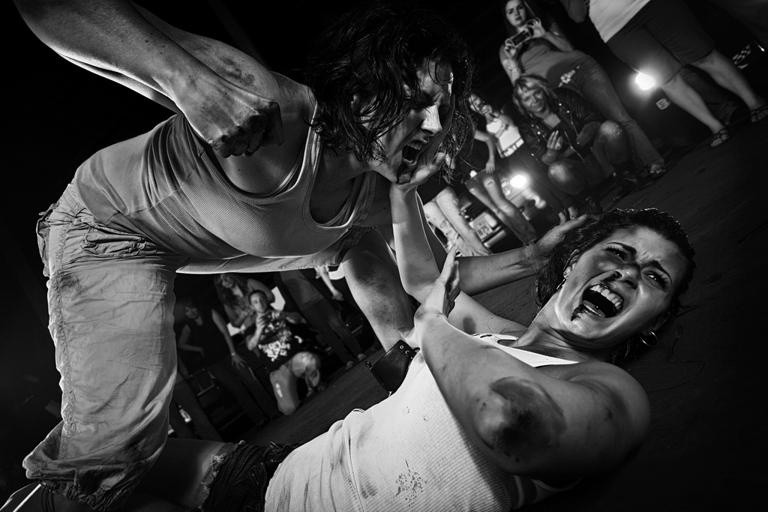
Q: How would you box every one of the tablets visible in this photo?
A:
[546,122,576,154]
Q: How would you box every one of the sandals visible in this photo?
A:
[748,102,768,126]
[709,124,733,149]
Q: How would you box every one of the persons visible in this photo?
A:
[496,0,668,176]
[2,0,599,510]
[512,73,665,216]
[562,0,767,147]
[415,178,492,256]
[445,129,537,246]
[465,91,579,223]
[126,123,698,512]
[168,271,369,441]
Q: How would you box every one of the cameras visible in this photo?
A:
[478,99,488,111]
[261,311,272,320]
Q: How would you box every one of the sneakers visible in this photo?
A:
[343,353,368,372]
[582,169,642,214]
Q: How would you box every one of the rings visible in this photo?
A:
[556,143,561,147]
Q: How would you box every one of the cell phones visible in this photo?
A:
[509,27,532,48]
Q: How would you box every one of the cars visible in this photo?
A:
[434,168,547,262]
[620,18,768,154]
